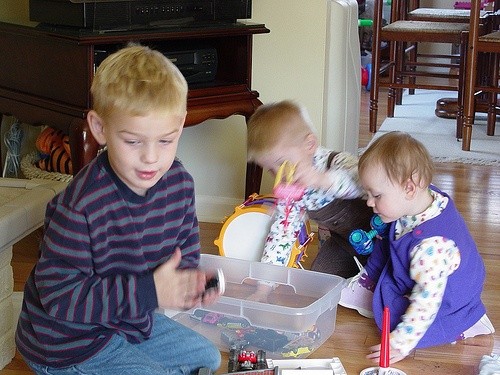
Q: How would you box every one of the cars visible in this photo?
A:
[227,341,268,372]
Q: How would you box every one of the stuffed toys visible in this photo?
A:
[34,124,73,175]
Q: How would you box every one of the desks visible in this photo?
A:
[0,18,270,201]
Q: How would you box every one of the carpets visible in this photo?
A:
[360,87,500,165]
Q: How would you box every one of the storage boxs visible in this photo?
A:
[164,254,348,361]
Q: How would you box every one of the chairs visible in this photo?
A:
[369,0,500,151]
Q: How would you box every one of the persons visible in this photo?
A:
[14,46,221,374]
[326,130,495,363]
[246,99,375,301]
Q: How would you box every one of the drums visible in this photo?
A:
[214,193,315,269]
